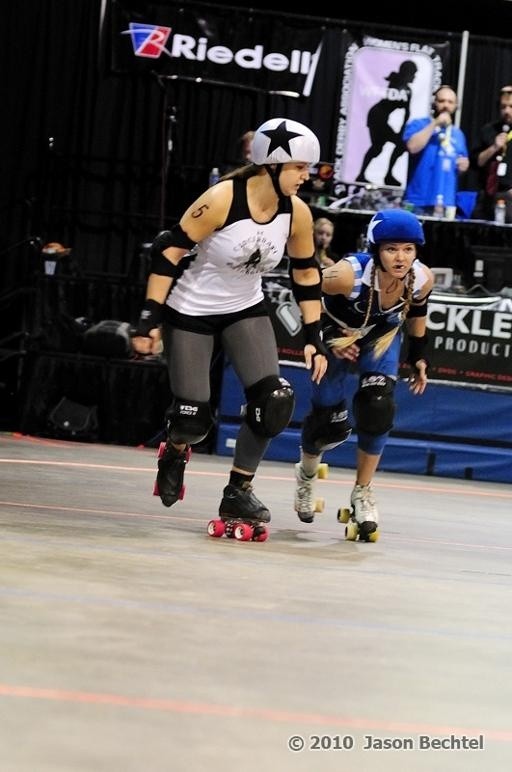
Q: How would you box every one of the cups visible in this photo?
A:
[445,206,457,219]
[44,248,57,275]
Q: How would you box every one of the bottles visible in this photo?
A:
[432,195,444,218]
[494,199,505,224]
[210,168,221,187]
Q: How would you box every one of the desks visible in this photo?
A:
[310,201,512,292]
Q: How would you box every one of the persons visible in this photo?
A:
[125,114,334,523]
[220,129,258,180]
[352,57,419,190]
[400,83,470,220]
[313,216,341,271]
[473,83,512,222]
[292,204,436,535]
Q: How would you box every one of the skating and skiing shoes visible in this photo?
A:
[151,440,192,507]
[294,458,328,522]
[206,484,271,542]
[336,483,381,542]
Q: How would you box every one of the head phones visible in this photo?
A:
[431,86,459,112]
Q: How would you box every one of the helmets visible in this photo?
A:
[249,115,322,165]
[367,208,426,246]
